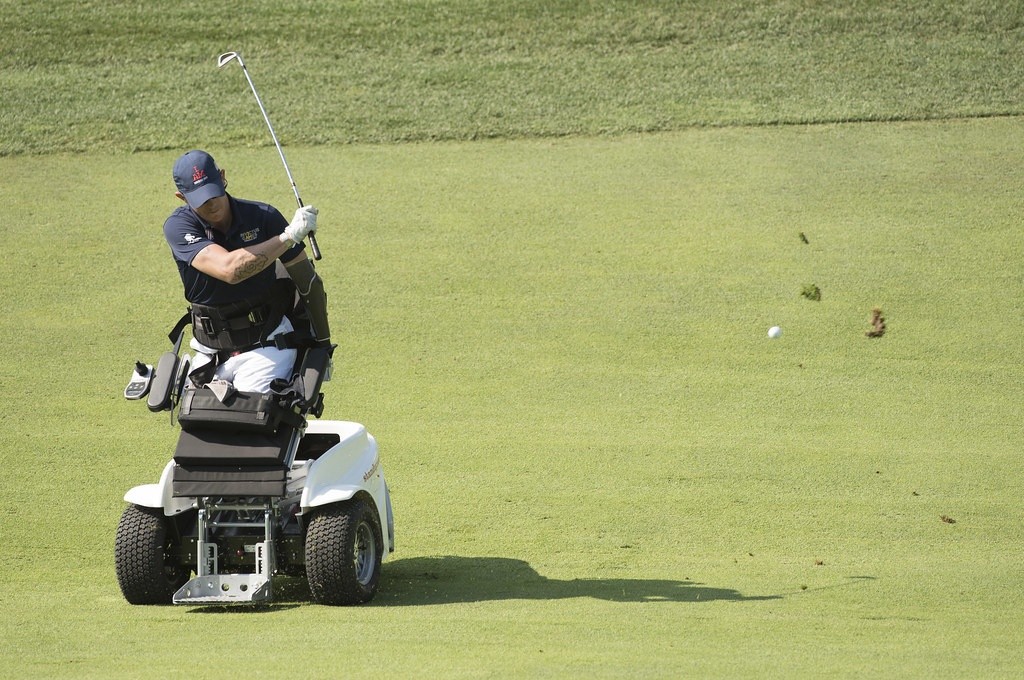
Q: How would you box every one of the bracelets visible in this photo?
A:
[315,337,330,342]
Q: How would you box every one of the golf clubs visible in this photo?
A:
[216,51,325,262]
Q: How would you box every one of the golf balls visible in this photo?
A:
[767,325,783,339]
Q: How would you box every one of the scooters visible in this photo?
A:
[113,270,396,606]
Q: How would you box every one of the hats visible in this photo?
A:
[173,150,225,209]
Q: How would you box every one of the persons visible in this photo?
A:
[163,150,331,394]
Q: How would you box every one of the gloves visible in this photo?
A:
[285,205,319,245]
